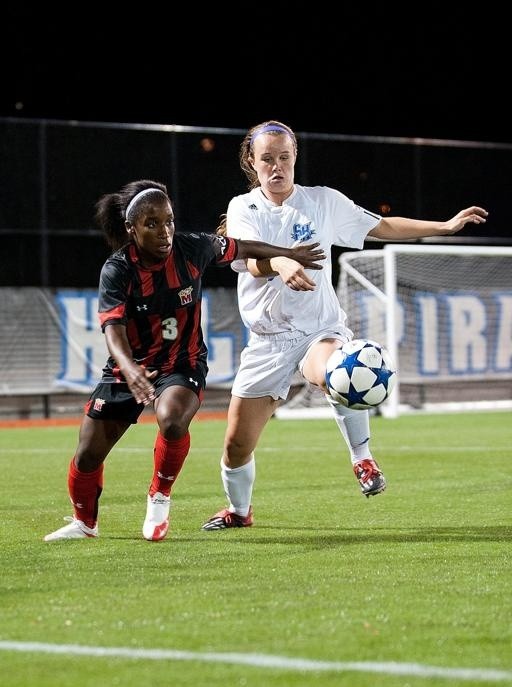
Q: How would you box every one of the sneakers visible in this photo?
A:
[44,518,98,541]
[352,459,386,497]
[142,491,170,541]
[203,504,253,530]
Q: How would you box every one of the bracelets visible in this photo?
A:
[255,254,278,276]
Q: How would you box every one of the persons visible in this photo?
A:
[198,119,491,532]
[41,178,327,540]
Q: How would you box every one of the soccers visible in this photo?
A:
[325,340,396,409]
